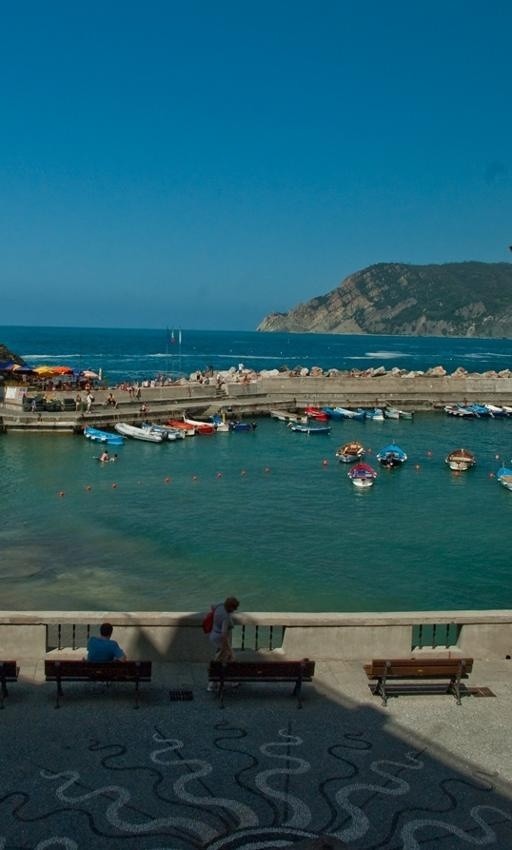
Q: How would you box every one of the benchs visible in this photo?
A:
[41,657,153,709]
[0,659,19,707]
[203,660,315,710]
[361,655,473,706]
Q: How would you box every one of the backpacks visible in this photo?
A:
[202,603,224,633]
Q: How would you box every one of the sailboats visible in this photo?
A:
[179,326,182,345]
[170,327,175,344]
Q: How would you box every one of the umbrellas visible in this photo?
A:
[0,359,102,380]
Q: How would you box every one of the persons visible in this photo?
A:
[206,597,241,693]
[86,621,128,666]
[100,450,111,463]
[109,453,120,464]
[45,369,251,391]
[85,389,94,414]
[76,393,82,410]
[104,392,120,410]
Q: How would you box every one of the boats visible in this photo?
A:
[495,466,512,491]
[444,448,476,473]
[347,455,379,490]
[207,414,257,433]
[334,440,365,465]
[264,403,417,425]
[287,421,333,435]
[112,415,219,445]
[375,439,408,470]
[441,403,512,419]
[81,423,126,447]
